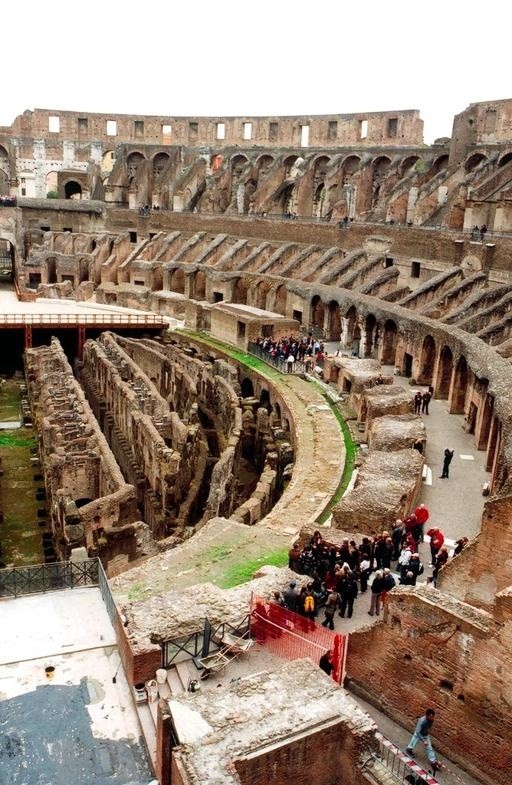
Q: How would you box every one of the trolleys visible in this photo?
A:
[197,634,262,681]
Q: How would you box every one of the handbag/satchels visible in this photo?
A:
[418,563,424,575]
[325,607,334,616]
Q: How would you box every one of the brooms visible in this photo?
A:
[113,642,127,683]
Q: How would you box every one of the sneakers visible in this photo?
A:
[358,591,364,593]
[407,748,415,758]
[302,622,334,633]
[368,610,379,616]
[428,561,433,568]
[432,760,442,770]
[339,612,352,618]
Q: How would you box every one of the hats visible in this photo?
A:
[411,553,419,557]
[290,579,296,588]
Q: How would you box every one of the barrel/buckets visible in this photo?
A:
[45,667,55,677]
[155,668,168,684]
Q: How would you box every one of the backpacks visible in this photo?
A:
[303,591,315,613]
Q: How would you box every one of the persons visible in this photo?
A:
[439,448,454,478]
[253,503,469,645]
[414,392,421,415]
[404,708,442,771]
[423,392,431,415]
[143,204,150,216]
[319,650,335,676]
[480,224,487,240]
[257,333,327,373]
[377,374,383,384]
